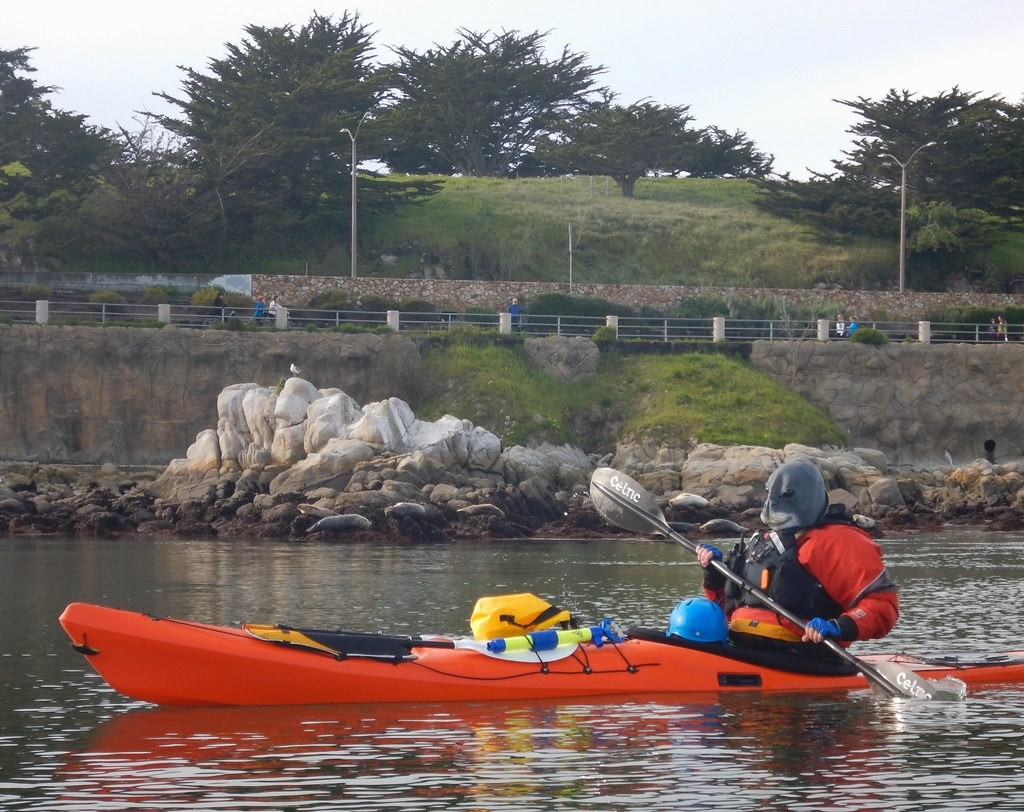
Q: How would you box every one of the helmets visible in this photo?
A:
[259,296,264,301]
[666,597,730,644]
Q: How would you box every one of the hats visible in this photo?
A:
[217,291,223,297]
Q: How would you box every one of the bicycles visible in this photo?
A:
[204,306,237,324]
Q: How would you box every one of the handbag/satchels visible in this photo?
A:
[469,593,582,640]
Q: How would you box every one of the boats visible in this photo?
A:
[59,603,1024,698]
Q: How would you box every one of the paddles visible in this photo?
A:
[588,465,941,701]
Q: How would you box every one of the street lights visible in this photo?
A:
[878,142,937,293]
[340,112,372,278]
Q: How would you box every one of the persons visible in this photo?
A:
[507,298,520,322]
[254,296,267,326]
[696,463,899,676]
[214,293,231,315]
[990,316,1008,341]
[836,314,845,336]
[268,296,282,318]
[846,316,858,336]
